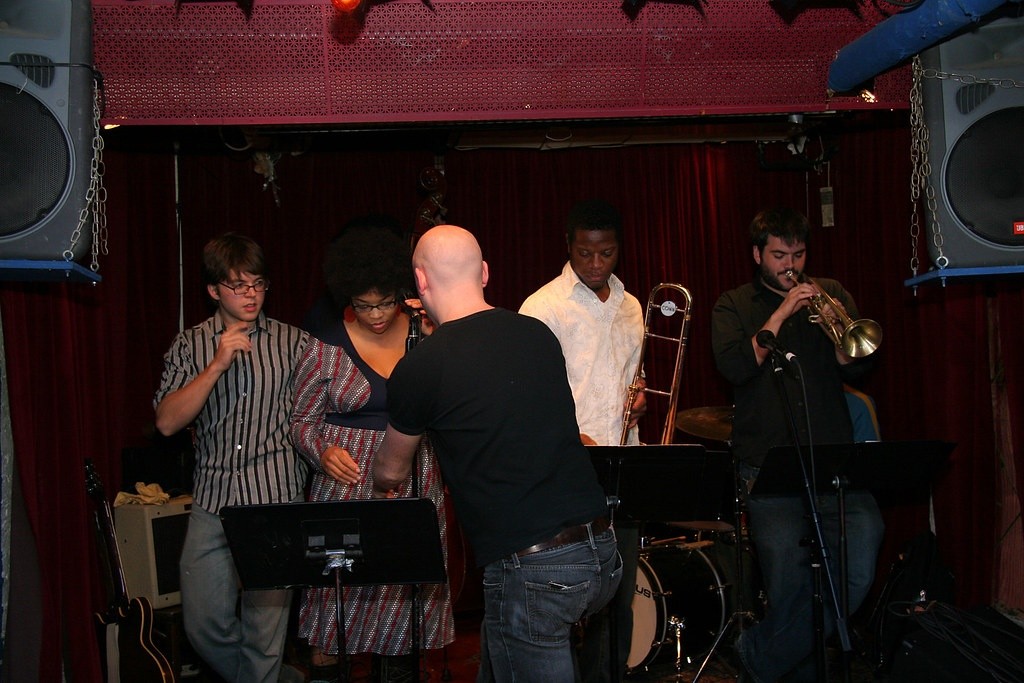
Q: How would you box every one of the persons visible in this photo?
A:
[153,229,310,683]
[711,208,887,683]
[290,226,456,683]
[518,199,648,683]
[372,224,623,683]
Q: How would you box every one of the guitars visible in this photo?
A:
[81,459,174,682]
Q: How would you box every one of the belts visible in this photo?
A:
[515,518,608,558]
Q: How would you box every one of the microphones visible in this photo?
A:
[397,287,423,322]
[755,330,798,362]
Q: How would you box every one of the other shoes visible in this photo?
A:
[733,637,754,683]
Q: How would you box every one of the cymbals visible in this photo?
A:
[675,404,737,442]
[621,537,731,674]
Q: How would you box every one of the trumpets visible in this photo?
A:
[779,269,883,359]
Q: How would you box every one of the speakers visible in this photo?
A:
[918,17,1024,270]
[114,494,193,611]
[0,0,96,264]
[893,602,1024,683]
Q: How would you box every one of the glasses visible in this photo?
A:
[218,279,269,295]
[351,297,397,313]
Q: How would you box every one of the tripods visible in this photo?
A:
[692,460,764,683]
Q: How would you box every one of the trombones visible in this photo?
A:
[620,282,693,449]
